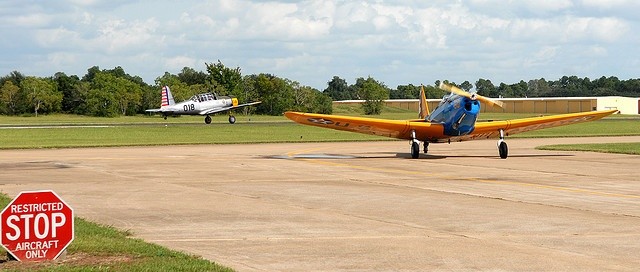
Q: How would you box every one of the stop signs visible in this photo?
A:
[0,190,74,263]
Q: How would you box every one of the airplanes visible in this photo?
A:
[145,86,261,124]
[283,84,619,159]
